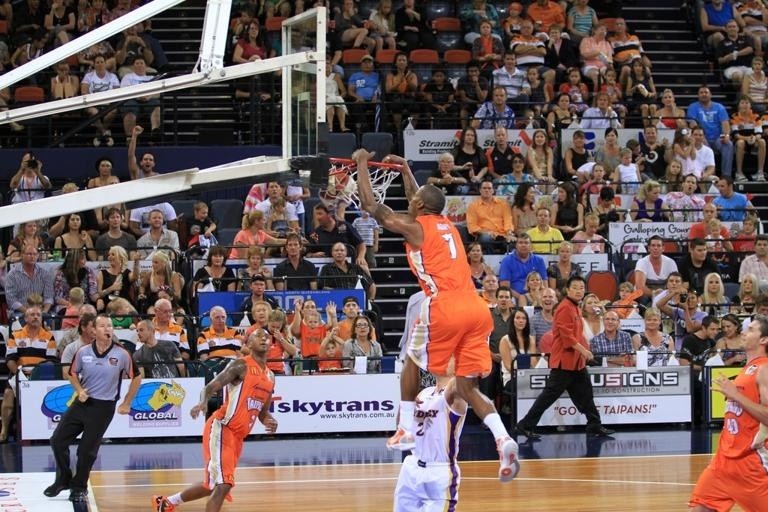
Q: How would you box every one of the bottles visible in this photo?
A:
[54,130,64,148]
[599,51,608,64]
[115,274,123,295]
[295,350,303,375]
[39,247,59,261]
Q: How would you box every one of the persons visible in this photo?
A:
[1,1,767,441]
[350,146,520,483]
[44,313,142,512]
[389,367,468,512]
[687,313,767,511]
[151,328,278,512]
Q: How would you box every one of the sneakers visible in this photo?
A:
[0,432,10,443]
[151,494,175,512]
[497,436,521,485]
[385,427,416,451]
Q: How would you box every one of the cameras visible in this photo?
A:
[26,152,38,169]
[679,293,687,302]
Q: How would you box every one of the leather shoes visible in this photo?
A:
[68,490,87,502]
[510,421,542,439]
[43,481,65,497]
[518,438,542,454]
[585,425,616,437]
[587,435,616,444]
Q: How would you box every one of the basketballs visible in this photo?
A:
[319,169,357,209]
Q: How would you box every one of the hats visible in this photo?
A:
[342,296,359,306]
[251,273,265,282]
